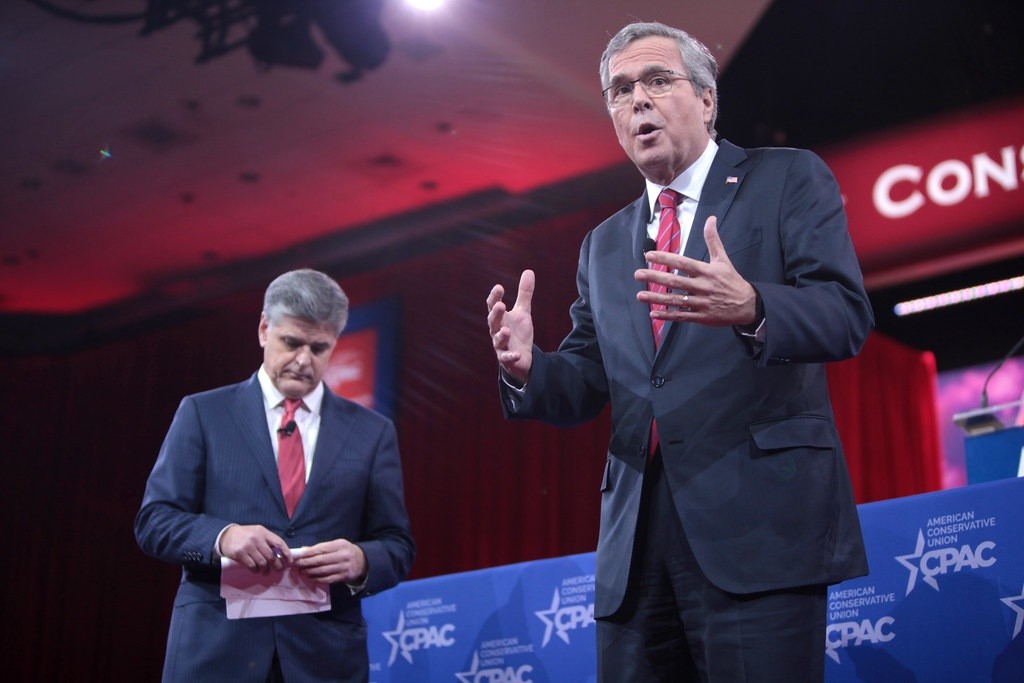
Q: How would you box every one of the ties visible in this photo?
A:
[277,398,306,521]
[646,189,681,461]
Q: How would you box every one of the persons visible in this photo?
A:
[484,19,877,683]
[133,268,413,683]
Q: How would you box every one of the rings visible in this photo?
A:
[682,295,689,309]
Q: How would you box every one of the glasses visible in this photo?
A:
[602,70,697,108]
